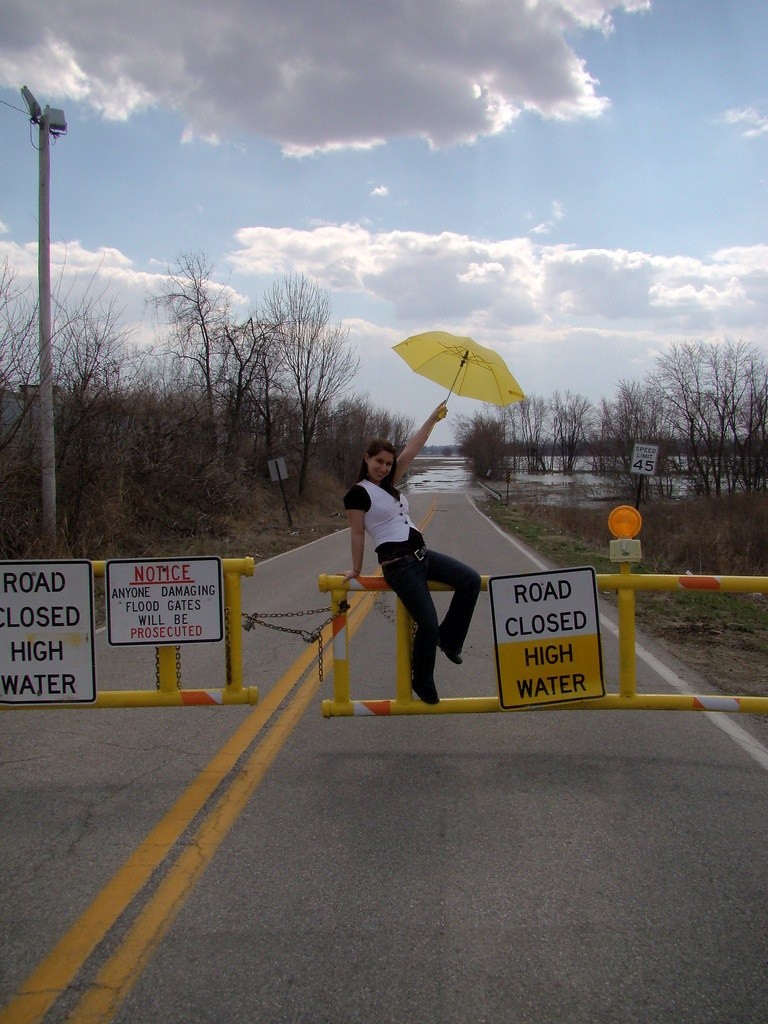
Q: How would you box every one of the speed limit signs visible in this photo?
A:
[630,443,659,477]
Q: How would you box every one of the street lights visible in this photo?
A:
[19,84,71,547]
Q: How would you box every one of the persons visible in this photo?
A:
[337,400,481,704]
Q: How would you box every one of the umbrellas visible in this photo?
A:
[391,331,525,421]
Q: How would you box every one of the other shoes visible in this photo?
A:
[436,631,462,665]
[411,680,439,704]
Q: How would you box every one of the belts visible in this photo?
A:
[383,546,427,572]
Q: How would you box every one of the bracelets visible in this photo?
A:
[353,569,360,574]
